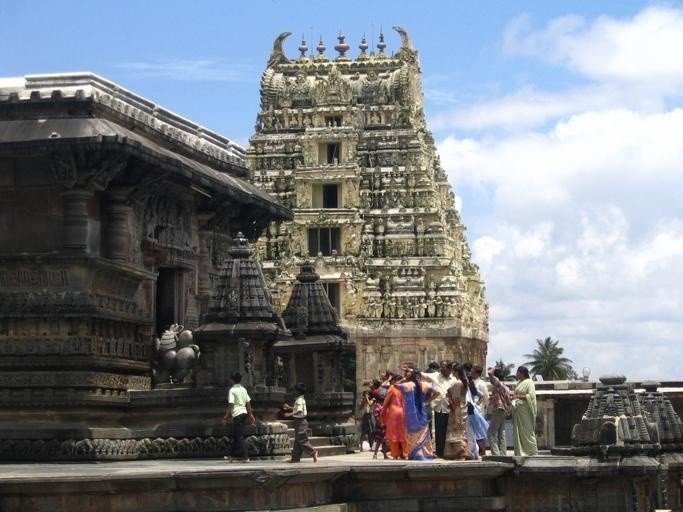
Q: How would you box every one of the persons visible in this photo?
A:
[221,372,255,463]
[358,359,537,461]
[283,383,318,463]
[363,296,457,318]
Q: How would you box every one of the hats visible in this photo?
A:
[374,378,381,385]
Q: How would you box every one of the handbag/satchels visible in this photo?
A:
[467,401,475,415]
